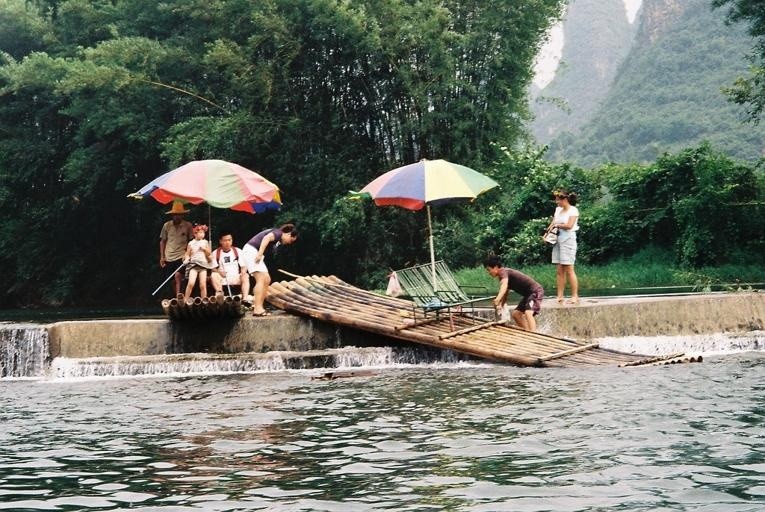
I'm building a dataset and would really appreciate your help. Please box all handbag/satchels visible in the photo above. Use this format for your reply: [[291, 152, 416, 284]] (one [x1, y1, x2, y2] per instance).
[[545, 226, 559, 245]]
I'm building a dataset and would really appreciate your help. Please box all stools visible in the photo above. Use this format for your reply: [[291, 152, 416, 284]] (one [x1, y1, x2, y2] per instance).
[[184, 277, 215, 298]]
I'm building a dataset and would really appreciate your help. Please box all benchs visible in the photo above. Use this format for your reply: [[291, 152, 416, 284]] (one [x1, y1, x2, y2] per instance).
[[394, 258, 502, 341]]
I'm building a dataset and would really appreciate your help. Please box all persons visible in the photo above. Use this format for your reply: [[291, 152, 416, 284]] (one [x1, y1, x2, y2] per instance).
[[482, 254, 545, 333], [209, 232, 252, 301], [543, 188, 581, 305], [184, 223, 211, 302], [159, 202, 194, 297], [241, 223, 300, 317]]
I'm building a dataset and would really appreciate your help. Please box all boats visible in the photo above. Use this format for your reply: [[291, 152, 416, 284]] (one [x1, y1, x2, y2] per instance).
[[157, 291, 263, 316], [262, 271, 704, 370]]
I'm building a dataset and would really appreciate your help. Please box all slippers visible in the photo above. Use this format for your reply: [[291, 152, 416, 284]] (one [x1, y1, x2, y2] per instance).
[[253, 310, 272, 317]]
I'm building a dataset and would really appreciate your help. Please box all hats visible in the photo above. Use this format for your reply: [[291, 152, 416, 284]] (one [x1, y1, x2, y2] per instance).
[[165, 202, 189, 214]]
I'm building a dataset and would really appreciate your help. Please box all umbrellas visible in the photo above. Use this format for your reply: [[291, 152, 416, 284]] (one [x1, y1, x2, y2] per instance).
[[127, 157, 283, 242], [345, 157, 501, 291]]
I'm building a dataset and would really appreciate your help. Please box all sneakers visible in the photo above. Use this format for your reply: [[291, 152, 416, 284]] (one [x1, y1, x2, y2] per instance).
[[557, 298, 579, 305]]
